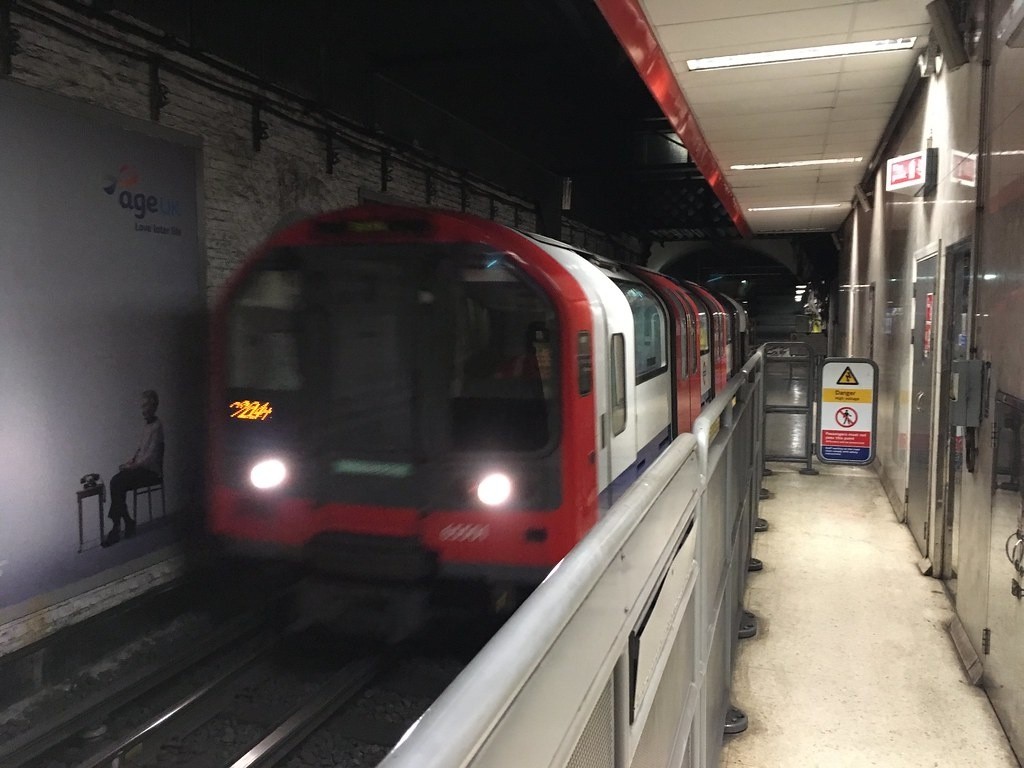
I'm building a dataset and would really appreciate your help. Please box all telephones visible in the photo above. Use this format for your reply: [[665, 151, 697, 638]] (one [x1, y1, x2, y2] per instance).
[[80, 473, 100, 489]]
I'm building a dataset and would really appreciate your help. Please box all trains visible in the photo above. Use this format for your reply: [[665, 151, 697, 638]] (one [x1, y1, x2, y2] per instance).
[[203, 199, 748, 626]]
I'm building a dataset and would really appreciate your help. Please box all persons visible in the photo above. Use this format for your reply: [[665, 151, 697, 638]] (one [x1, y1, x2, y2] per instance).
[[100, 389, 164, 548]]
